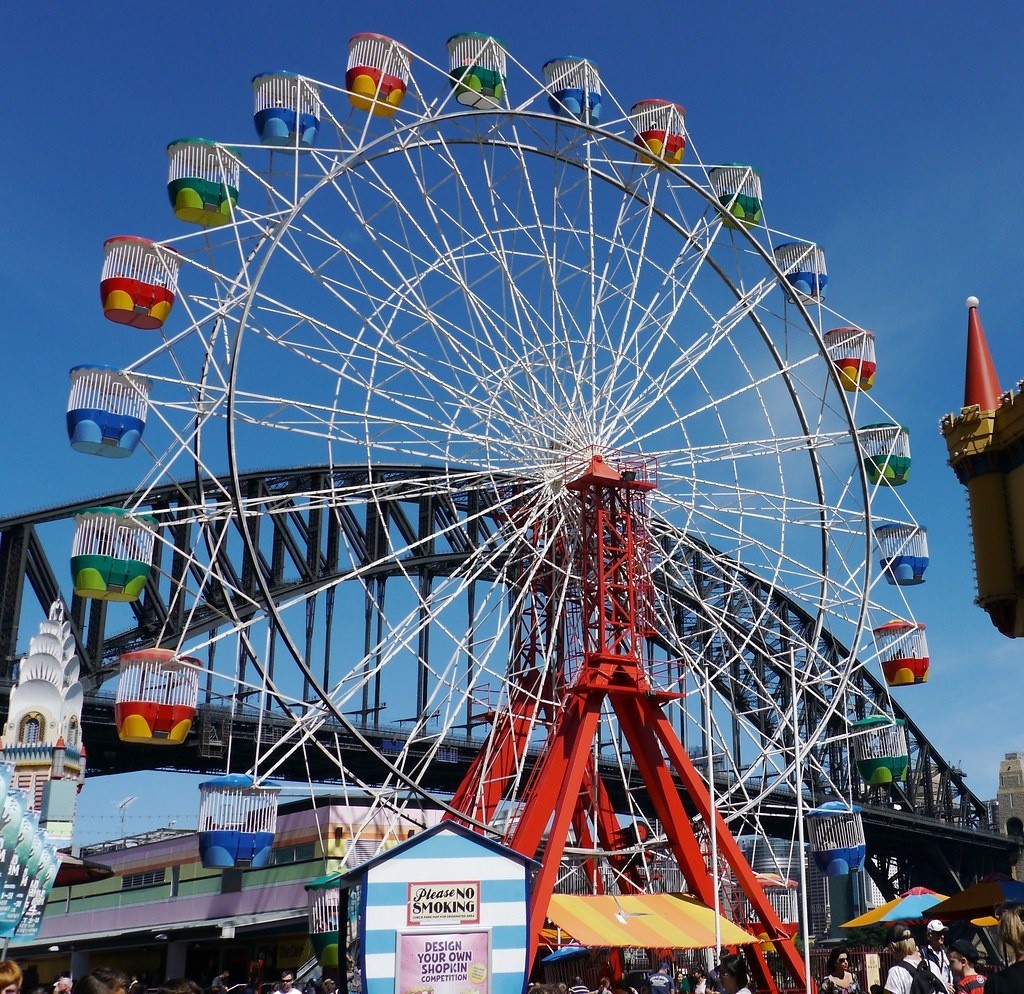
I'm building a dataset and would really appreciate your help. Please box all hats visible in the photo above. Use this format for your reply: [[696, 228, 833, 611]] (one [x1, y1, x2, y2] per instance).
[[659, 963, 668, 968], [950, 939, 979, 962], [883, 924, 913, 946], [927, 920, 948, 936]]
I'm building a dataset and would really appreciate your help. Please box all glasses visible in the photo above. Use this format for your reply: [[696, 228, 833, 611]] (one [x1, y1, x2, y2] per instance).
[[934, 931, 944, 936], [839, 958, 849, 963], [718, 971, 728, 978]]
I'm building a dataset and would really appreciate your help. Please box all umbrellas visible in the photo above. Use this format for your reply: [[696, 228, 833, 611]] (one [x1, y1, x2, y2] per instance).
[[838, 870, 1024, 968]]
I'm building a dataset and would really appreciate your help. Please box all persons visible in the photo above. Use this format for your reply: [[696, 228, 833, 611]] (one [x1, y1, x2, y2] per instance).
[[205, 817, 221, 830], [882, 918, 988, 994], [818, 945, 862, 994], [891, 647, 918, 660], [983, 906, 1024, 994], [0, 943, 363, 994], [529, 948, 756, 994]]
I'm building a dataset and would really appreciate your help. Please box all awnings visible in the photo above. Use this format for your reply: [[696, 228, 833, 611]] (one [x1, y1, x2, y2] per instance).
[[538, 889, 790, 962], [755, 873, 798, 891]]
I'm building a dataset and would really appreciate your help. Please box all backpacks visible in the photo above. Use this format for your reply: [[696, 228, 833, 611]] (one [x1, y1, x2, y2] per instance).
[[897, 956, 948, 994]]
